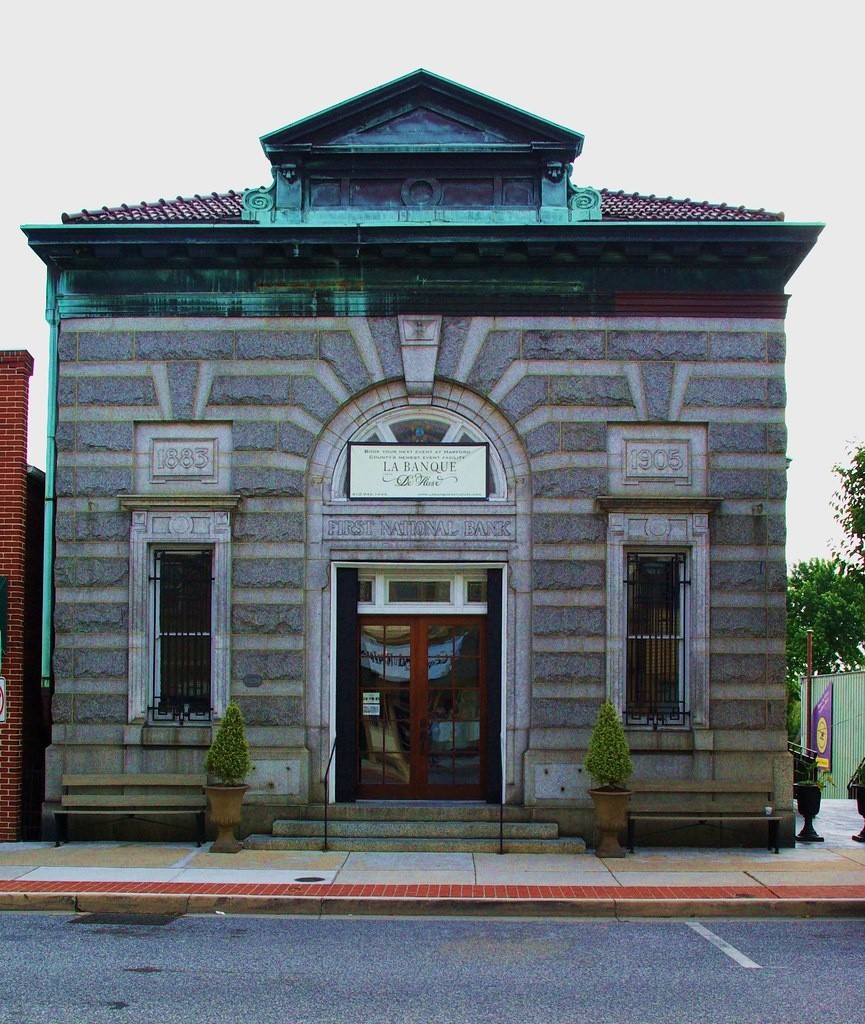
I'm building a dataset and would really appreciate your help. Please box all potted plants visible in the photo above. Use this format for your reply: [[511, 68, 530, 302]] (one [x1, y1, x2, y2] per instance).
[[852, 766, 865, 842], [202, 699, 250, 854], [584, 694, 634, 858], [792, 762, 836, 841]]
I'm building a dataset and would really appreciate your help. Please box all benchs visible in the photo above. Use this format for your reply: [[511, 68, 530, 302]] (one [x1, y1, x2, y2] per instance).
[[626, 781, 784, 854], [52, 773, 207, 847]]
